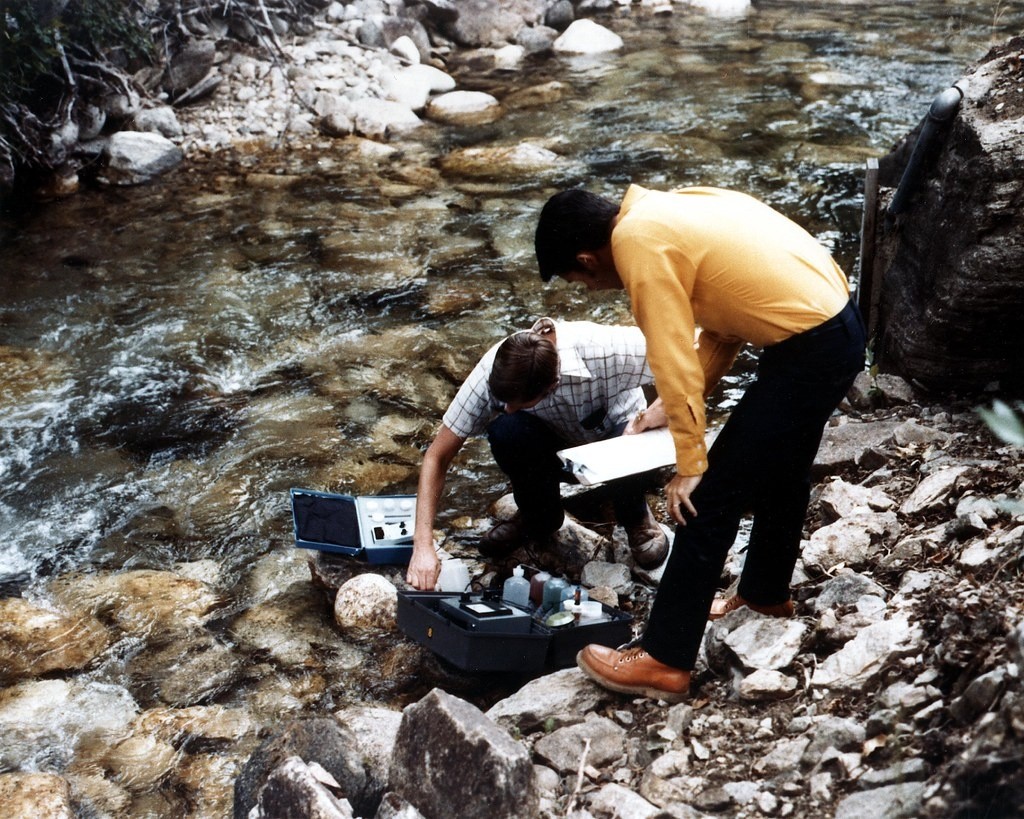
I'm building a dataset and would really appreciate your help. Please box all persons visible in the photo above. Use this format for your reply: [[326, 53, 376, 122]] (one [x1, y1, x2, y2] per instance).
[[531, 181, 865, 696], [407, 315, 670, 595]]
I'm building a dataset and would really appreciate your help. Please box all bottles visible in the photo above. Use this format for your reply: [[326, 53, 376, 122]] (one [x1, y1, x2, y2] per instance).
[[503, 568, 529, 605], [531, 568, 551, 603], [543, 573, 570, 612], [560, 577, 588, 613]]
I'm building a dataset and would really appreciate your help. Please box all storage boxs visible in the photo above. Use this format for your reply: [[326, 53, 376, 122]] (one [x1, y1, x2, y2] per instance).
[[398, 562, 635, 688], [290, 487, 416, 564]]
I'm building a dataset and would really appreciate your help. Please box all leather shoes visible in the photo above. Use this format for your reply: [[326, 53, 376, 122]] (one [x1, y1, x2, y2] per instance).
[[710, 595, 794, 621], [575, 643, 692, 705]]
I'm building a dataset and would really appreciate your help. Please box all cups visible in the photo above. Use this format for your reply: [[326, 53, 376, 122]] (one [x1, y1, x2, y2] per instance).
[[564, 600, 601, 617]]
